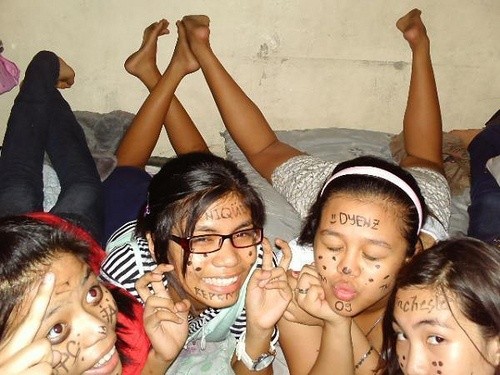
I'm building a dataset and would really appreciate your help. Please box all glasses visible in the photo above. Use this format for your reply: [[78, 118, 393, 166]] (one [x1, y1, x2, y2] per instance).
[[168, 223, 263, 254]]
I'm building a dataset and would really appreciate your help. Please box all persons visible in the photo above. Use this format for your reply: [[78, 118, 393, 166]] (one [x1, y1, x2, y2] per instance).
[[0, 49, 152, 375], [381, 99, 500, 375], [95, 19, 293, 375], [181, 7, 453, 375]]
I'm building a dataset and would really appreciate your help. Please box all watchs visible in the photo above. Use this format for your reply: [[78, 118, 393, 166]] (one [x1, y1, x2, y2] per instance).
[[235, 330, 278, 371]]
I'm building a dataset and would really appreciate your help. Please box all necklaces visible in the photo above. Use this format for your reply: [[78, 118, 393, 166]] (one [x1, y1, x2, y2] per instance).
[[364, 309, 385, 337]]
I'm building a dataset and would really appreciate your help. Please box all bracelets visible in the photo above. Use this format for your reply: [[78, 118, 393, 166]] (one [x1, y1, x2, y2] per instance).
[[353, 346, 374, 372]]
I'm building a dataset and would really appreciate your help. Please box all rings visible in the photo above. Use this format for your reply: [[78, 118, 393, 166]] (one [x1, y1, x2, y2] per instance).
[[297, 288, 309, 294]]
